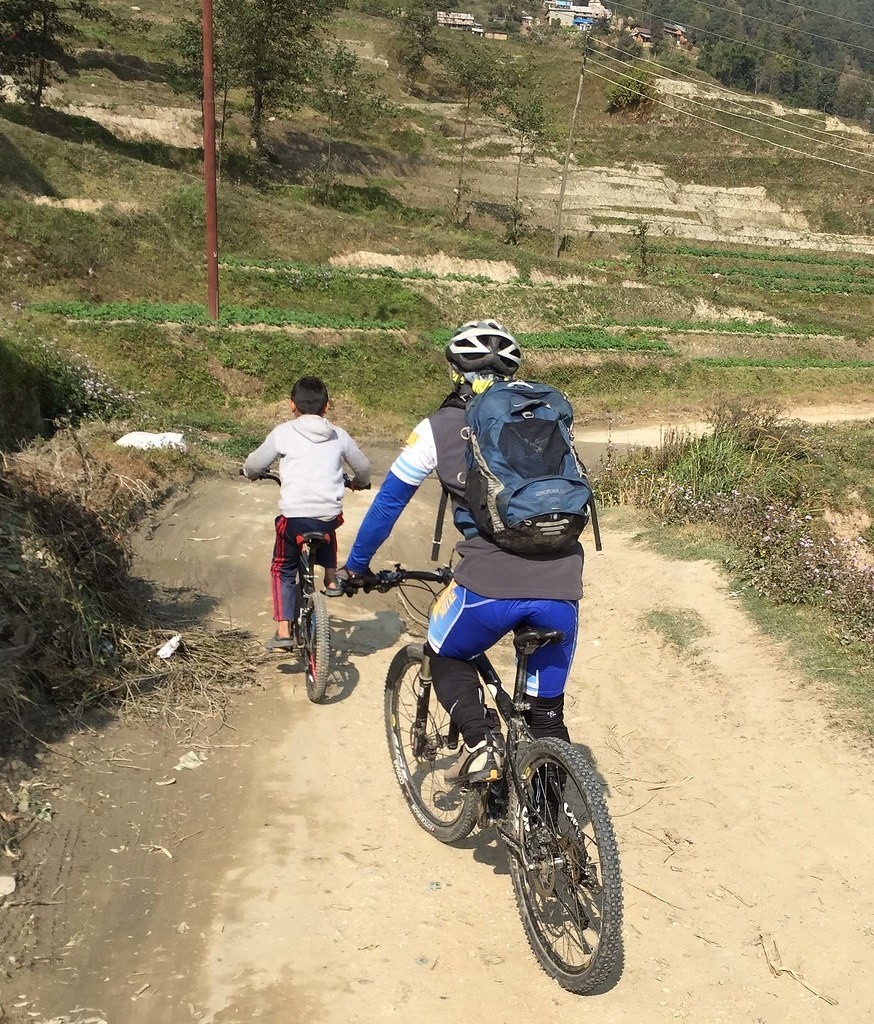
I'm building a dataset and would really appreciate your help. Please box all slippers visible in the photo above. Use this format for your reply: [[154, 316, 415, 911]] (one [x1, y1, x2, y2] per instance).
[[272, 630, 295, 647], [324, 576, 344, 597]]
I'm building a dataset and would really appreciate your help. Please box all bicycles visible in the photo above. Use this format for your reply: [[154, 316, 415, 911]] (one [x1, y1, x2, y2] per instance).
[[239, 469, 371, 705], [323, 570, 625, 997]]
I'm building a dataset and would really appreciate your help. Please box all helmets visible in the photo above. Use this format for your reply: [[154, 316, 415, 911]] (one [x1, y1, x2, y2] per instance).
[[445, 318, 522, 375]]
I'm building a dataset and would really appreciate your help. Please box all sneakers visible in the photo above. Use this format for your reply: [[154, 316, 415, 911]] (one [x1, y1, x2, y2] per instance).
[[444, 730, 503, 787]]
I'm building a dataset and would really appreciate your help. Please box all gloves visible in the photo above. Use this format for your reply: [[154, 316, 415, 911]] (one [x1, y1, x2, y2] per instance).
[[336, 565, 376, 599]]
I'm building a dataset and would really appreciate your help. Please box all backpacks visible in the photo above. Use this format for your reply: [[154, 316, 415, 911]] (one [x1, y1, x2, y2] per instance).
[[436, 379, 593, 558]]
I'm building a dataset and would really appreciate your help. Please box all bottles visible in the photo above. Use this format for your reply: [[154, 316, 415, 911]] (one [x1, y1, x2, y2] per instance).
[[486, 708, 506, 770]]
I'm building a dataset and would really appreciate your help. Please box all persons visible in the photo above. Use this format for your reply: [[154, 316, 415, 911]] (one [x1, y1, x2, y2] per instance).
[[242, 377, 370, 648], [334, 320, 584, 859]]
